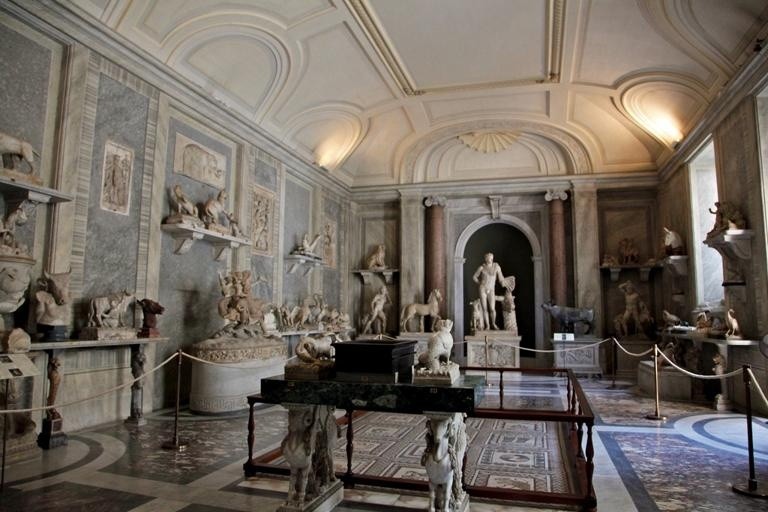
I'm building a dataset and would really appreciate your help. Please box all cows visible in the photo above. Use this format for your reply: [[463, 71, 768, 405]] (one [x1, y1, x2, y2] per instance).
[[541, 298, 597, 335], [86, 286, 135, 329], [35, 264, 73, 327]]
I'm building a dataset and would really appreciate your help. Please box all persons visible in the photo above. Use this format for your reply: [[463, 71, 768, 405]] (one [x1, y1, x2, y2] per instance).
[[203, 191, 232, 224], [619, 280, 642, 337], [362, 286, 392, 335], [473, 253, 505, 330], [709, 202, 722, 230], [175, 185, 200, 218]]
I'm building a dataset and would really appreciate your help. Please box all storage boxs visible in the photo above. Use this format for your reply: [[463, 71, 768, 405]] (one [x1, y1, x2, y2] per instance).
[[329, 337, 418, 374]]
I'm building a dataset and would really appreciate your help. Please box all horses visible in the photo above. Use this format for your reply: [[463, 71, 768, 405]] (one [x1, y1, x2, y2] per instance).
[[400, 287, 443, 332]]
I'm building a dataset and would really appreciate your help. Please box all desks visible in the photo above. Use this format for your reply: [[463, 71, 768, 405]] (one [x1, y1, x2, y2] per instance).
[[241, 372, 486, 496], [23, 332, 170, 450]]
